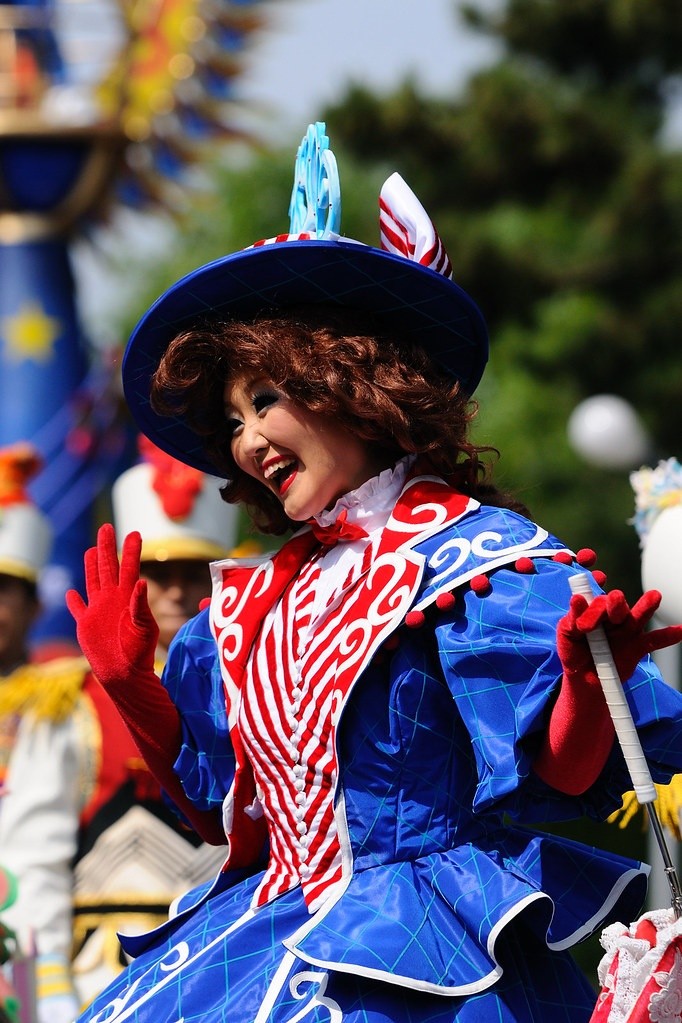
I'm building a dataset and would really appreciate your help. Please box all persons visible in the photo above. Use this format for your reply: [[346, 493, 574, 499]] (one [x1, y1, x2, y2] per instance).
[[65, 312, 681, 1023], [0, 462, 242, 1023]]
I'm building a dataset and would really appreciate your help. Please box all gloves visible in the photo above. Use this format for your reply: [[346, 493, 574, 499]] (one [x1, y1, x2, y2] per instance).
[[66, 524, 184, 754], [527, 590, 681, 796]]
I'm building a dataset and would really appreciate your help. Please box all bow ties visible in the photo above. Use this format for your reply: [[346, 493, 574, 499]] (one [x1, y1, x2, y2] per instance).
[[305, 508, 369, 545]]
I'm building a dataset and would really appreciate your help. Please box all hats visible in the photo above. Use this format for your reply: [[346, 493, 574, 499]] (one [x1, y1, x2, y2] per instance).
[[0, 440, 57, 581], [112, 461, 238, 559], [121, 122, 490, 481]]
